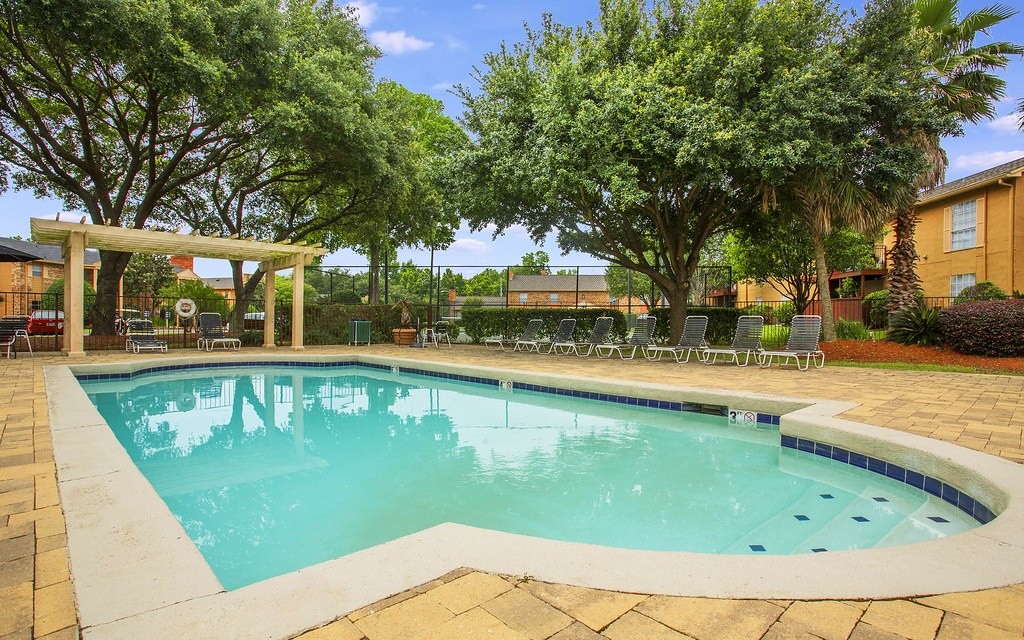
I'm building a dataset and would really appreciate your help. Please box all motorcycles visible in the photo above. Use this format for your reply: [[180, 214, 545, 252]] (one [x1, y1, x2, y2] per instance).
[[114, 313, 131, 335]]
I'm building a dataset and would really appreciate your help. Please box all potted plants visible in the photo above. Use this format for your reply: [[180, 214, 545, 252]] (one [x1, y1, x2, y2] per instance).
[[389, 299, 417, 344], [835, 278, 861, 298]]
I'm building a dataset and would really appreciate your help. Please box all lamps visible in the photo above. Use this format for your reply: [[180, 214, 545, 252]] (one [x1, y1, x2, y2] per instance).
[[582, 294, 585, 299]]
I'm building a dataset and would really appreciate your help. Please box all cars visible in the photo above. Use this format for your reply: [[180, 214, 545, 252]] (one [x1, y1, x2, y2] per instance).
[[27, 310, 64, 337]]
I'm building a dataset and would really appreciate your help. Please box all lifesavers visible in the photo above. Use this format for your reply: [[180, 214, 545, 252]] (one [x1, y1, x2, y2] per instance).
[[176, 299, 196, 317]]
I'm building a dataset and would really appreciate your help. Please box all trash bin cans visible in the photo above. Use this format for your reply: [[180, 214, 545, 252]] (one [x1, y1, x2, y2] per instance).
[[349, 319, 371, 347]]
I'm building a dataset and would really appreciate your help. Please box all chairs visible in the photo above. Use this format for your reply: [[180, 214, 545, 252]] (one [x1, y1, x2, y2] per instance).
[[0, 316, 34, 360], [758, 315, 825, 371], [126, 318, 169, 355], [486, 318, 543, 353], [648, 316, 710, 363], [554, 317, 614, 356], [513, 319, 576, 353], [595, 316, 658, 360], [197, 311, 242, 352], [702, 315, 766, 367], [422, 320, 452, 349]]
